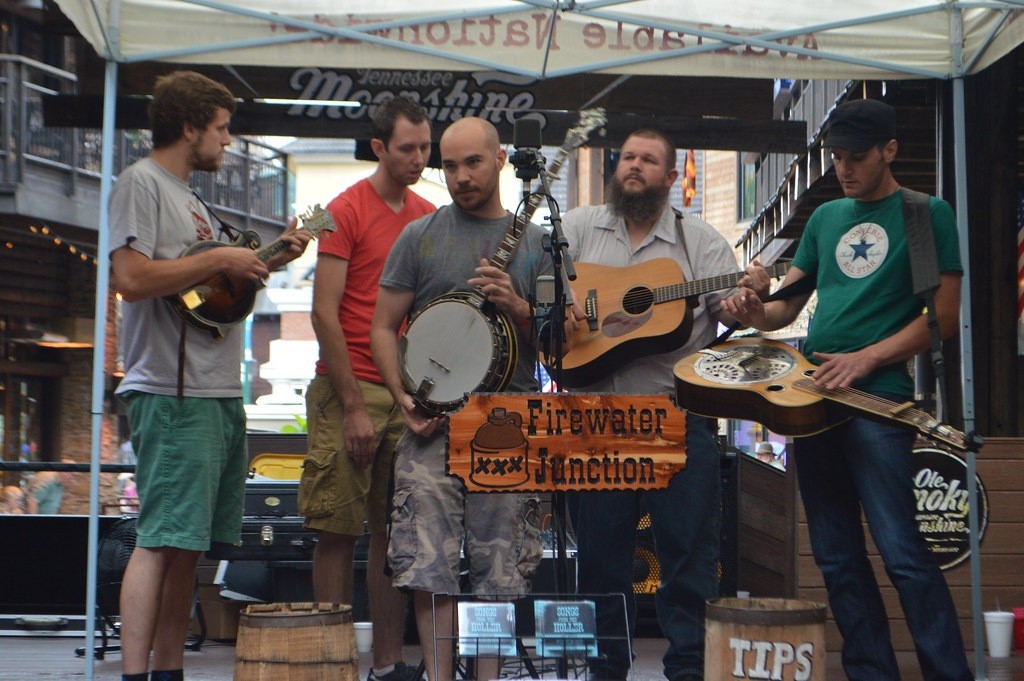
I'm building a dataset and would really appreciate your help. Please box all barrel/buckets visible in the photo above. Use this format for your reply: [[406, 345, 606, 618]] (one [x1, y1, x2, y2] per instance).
[[704, 598, 827, 681], [233, 602, 360, 681]]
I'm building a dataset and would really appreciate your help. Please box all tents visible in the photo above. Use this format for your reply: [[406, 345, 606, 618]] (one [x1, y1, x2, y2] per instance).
[[46, 0, 1023, 681]]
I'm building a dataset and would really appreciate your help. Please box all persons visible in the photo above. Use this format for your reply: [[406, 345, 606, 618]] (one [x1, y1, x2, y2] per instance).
[[109, 73, 313, 681], [551, 127, 770, 681], [367, 116, 574, 681], [719, 98, 975, 681], [0, 459, 139, 515], [297, 95, 439, 680]]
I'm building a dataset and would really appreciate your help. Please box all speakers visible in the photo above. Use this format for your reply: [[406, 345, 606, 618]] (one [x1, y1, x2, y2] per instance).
[[632, 451, 738, 637]]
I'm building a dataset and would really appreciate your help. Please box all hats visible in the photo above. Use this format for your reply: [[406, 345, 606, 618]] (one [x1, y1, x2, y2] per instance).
[[818, 99, 899, 153]]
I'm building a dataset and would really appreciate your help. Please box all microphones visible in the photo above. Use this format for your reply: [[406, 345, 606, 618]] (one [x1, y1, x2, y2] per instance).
[[514, 118, 542, 203]]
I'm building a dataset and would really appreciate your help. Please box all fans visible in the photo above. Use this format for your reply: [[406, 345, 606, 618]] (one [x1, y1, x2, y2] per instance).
[[76, 517, 207, 660]]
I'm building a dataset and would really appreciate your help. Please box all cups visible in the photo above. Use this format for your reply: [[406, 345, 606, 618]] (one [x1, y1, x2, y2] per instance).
[[1013, 609, 1024, 654], [984, 611, 1015, 658]]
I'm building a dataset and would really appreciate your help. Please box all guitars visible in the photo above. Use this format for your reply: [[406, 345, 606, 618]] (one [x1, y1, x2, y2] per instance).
[[671, 336, 984, 455], [539, 256, 791, 387], [168, 203, 339, 340], [398, 108, 608, 417]]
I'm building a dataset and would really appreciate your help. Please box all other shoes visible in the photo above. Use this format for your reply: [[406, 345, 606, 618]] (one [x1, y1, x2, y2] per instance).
[[367, 662, 426, 681]]
